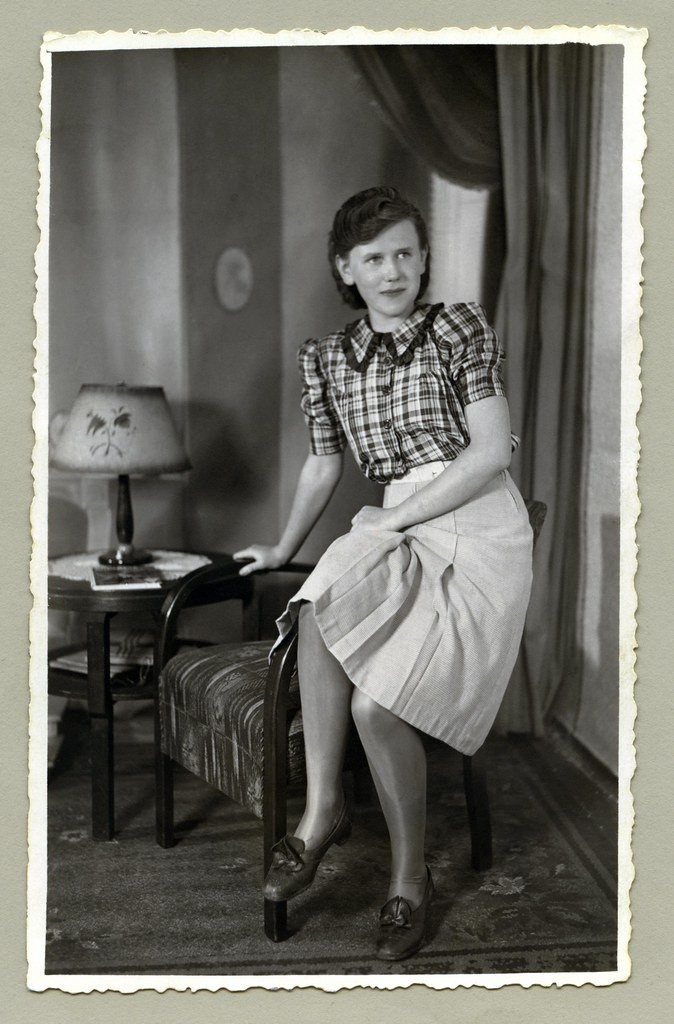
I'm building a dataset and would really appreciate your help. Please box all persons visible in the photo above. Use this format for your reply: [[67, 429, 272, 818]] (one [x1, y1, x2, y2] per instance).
[[233, 185, 534, 959]]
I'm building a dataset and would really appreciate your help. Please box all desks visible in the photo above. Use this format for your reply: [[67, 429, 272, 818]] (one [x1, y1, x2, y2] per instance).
[[48, 546, 265, 845]]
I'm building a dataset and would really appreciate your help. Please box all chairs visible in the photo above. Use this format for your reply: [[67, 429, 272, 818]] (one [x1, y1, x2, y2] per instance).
[[153, 498, 548, 945]]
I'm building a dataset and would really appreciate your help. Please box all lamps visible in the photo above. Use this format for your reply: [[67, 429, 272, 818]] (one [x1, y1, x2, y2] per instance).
[[51, 380, 194, 568]]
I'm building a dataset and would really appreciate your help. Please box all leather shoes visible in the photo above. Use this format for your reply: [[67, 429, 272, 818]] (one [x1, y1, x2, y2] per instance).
[[376, 864, 436, 961], [262, 796, 356, 903]]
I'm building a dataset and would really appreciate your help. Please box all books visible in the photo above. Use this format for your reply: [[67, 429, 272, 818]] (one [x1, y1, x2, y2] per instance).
[[88, 565, 162, 590], [49, 645, 154, 678]]
